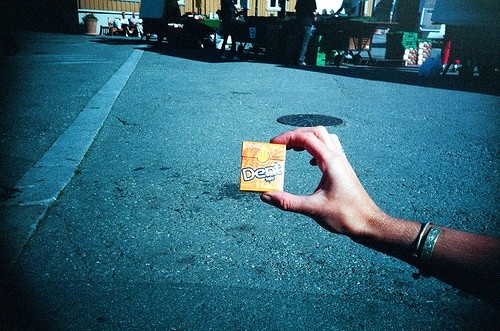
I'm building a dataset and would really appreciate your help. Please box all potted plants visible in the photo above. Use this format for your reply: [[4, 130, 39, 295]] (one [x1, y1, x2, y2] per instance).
[[82, 13, 98, 33]]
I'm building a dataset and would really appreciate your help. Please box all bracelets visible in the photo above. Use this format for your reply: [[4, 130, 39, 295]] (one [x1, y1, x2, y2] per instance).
[[419, 226, 442, 278], [412, 221, 433, 267]]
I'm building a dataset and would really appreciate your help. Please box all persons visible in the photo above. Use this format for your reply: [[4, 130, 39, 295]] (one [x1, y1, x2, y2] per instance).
[[294, 0, 317, 66], [259, 126, 500, 307], [217, 0, 241, 61], [121, 12, 133, 37], [129, 12, 144, 39]]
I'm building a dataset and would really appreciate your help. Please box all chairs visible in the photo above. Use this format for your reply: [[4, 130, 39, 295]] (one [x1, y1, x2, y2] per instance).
[[142, 18, 176, 52], [216, 10, 252, 53]]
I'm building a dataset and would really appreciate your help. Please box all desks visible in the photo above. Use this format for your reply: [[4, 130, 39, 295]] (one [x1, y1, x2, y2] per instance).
[[179, 17, 222, 50], [316, 14, 399, 66]]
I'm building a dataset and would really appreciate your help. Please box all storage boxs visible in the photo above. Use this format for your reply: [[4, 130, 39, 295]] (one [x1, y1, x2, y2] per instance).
[[239, 142, 288, 193], [386, 31, 420, 67], [417, 38, 432, 65]]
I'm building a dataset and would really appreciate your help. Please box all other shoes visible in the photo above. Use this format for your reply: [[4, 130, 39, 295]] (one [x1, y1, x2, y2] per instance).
[[440, 66, 445, 72], [234, 56, 240, 60], [451, 68, 457, 73], [221, 55, 228, 60], [296, 61, 310, 70]]
